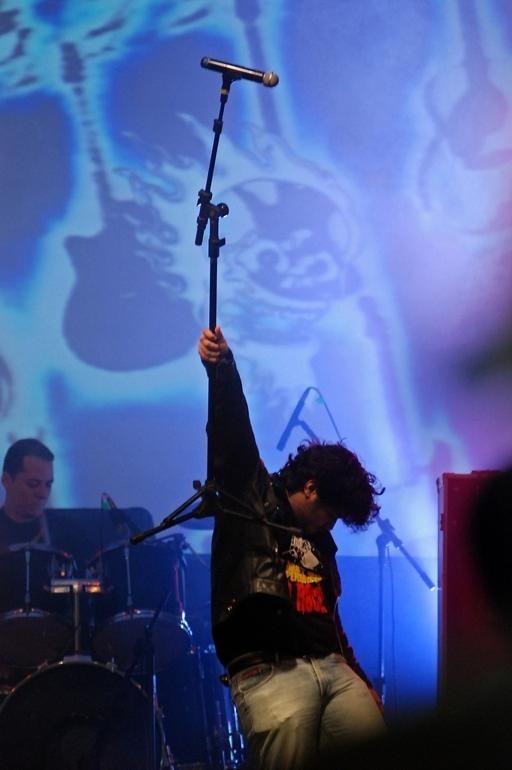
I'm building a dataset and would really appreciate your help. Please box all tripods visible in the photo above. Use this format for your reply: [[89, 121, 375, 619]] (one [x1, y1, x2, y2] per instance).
[[130, 78, 304, 545]]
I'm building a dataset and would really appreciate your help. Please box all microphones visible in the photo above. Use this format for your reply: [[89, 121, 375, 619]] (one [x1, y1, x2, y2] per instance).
[[277, 392, 308, 451], [200, 56, 279, 87], [106, 494, 128, 538]]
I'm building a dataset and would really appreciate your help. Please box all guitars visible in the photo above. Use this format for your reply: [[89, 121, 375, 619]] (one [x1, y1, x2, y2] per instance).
[[59, 40, 207, 371]]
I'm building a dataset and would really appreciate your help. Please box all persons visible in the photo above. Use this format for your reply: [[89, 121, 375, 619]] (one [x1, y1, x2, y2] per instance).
[[198, 325, 373, 770], [320, 468, 512, 770], [0, 439, 103, 613]]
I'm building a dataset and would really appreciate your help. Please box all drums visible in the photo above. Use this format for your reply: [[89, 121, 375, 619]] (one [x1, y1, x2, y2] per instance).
[[85, 538, 191, 674], [1, 655, 165, 769], [0, 542, 78, 668]]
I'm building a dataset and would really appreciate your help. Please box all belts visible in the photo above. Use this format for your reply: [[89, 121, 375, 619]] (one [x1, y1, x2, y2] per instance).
[[227, 652, 304, 678]]
